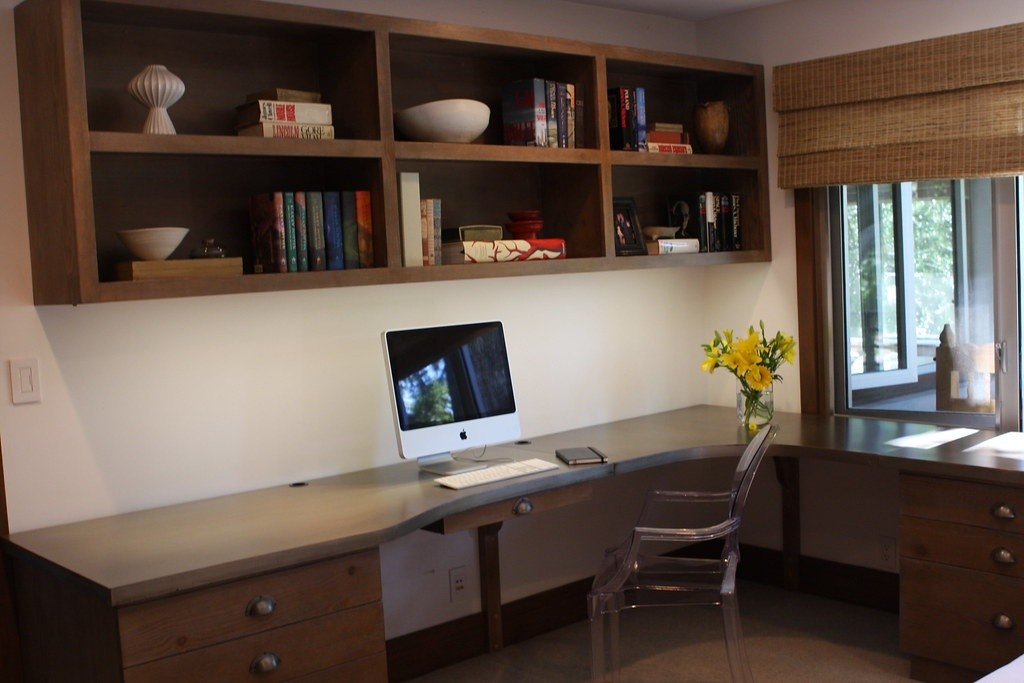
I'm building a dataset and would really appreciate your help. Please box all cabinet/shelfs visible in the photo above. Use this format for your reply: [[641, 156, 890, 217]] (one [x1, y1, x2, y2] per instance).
[[13, 0, 772, 305]]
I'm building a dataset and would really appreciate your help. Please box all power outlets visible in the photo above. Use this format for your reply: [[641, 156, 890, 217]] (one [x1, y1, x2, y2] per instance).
[[451, 566, 471, 604]]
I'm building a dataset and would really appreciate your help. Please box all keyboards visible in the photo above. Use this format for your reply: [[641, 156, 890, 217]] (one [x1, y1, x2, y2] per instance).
[[433, 458, 558, 491]]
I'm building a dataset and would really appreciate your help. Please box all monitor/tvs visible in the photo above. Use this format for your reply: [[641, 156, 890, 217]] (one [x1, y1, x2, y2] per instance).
[[381, 320, 522, 475]]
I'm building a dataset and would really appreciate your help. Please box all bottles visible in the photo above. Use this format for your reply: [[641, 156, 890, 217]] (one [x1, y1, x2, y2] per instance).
[[187, 238, 224, 258]]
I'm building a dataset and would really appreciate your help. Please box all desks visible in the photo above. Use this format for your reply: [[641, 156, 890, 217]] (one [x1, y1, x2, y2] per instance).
[[0, 405, 1024, 683]]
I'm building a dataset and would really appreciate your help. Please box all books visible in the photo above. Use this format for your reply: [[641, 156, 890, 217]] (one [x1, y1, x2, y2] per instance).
[[234, 99, 332, 128], [238, 122, 334, 140], [555, 446, 608, 465], [501, 78, 588, 147], [248, 184, 374, 273], [246, 88, 321, 104], [607, 87, 695, 153], [400, 172, 567, 267], [646, 191, 739, 256]]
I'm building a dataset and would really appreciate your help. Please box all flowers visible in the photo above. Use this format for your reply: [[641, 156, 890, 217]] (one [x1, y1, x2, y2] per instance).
[[701, 319, 797, 430]]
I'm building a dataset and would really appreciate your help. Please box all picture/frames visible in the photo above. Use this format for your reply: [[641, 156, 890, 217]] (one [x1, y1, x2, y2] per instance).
[[612, 196, 648, 256]]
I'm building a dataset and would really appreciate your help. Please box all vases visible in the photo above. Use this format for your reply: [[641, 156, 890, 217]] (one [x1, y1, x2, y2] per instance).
[[736, 378, 775, 424]]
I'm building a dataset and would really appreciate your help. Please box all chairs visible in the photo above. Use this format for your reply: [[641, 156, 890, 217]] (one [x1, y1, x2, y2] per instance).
[[588, 423, 779, 683]]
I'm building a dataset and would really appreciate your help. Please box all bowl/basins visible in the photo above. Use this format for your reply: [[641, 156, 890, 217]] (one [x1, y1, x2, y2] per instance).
[[119, 225, 190, 262], [396, 99, 492, 143]]
